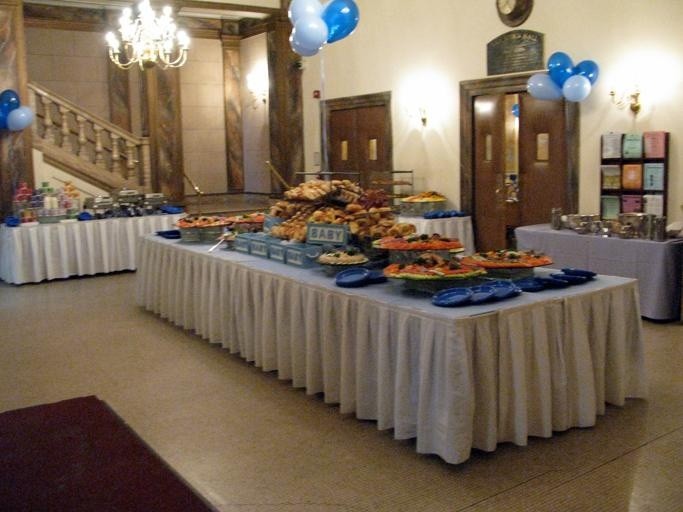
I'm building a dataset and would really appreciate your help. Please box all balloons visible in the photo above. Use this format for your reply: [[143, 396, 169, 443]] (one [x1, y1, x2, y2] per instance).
[[286, 1, 360, 57], [525, 73, 561, 100], [573, 60, 599, 87], [547, 51, 573, 87], [562, 74, 592, 102], [511, 104, 519, 117], [0, 90, 34, 132]]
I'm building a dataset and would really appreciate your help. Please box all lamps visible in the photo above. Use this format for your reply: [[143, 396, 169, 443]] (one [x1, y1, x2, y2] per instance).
[[606, 73, 640, 113], [102, 0, 193, 72]]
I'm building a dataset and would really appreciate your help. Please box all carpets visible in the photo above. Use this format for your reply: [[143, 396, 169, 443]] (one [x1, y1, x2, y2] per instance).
[[0, 394, 220, 512]]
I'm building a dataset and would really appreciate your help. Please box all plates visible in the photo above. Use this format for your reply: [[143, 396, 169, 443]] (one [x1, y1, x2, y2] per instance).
[[421, 208, 466, 219], [400, 197, 447, 204], [152, 215, 265, 239], [75, 203, 184, 221], [317, 233, 600, 309]]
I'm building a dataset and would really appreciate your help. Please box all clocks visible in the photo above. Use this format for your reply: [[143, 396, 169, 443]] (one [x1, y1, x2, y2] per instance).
[[496, 0, 533, 27]]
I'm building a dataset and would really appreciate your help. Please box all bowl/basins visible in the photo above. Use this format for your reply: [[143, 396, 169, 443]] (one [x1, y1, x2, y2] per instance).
[[34, 214, 66, 224], [548, 206, 681, 242], [4, 217, 19, 226]]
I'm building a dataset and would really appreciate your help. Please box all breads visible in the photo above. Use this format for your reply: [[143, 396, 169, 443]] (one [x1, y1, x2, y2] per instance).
[[269, 178, 417, 242]]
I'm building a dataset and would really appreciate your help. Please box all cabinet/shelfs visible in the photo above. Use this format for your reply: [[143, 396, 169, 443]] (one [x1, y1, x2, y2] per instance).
[[367, 169, 414, 209]]
